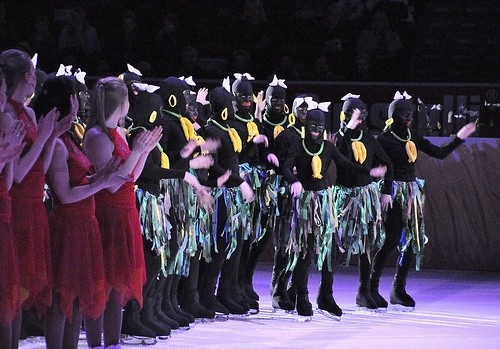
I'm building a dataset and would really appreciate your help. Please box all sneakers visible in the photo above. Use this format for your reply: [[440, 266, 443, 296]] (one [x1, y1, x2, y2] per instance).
[[118, 287, 261, 349], [272, 294, 295, 315], [295, 299, 313, 322], [354, 293, 377, 315], [316, 295, 343, 320], [389, 291, 415, 313], [370, 291, 389, 312]]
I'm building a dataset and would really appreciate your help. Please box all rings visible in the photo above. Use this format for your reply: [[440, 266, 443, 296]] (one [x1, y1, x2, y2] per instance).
[[15, 134, 19, 137]]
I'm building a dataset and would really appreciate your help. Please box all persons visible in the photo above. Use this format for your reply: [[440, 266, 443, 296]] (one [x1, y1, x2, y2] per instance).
[[34, 75, 134, 349], [82, 78, 163, 348], [372, 99, 477, 308], [31, 0, 416, 102], [0, 68, 26, 349], [121, 77, 394, 338], [0, 48, 76, 349]]
